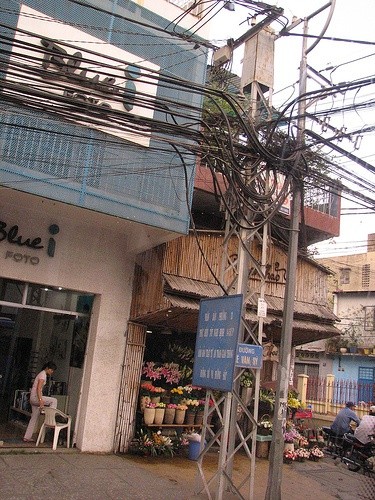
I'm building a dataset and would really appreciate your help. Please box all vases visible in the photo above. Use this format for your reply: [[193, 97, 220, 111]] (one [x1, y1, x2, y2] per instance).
[[284, 443, 294, 454], [164, 409, 175, 425], [154, 409, 165, 425], [144, 408, 155, 424], [260, 425, 270, 436], [195, 411, 204, 425], [175, 410, 185, 425], [184, 411, 196, 425], [242, 387, 253, 406]]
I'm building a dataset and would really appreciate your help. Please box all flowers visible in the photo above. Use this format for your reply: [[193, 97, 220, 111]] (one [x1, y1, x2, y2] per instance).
[[128, 343, 324, 464]]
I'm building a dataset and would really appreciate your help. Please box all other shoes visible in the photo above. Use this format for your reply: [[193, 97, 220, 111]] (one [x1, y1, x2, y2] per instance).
[[24, 438, 35, 442]]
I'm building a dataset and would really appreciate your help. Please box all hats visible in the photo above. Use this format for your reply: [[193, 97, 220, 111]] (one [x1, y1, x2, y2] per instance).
[[369, 405, 375, 413], [346, 401, 354, 407]]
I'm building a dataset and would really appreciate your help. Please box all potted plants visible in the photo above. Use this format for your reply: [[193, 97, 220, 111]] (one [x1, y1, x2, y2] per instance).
[[326, 337, 349, 354]]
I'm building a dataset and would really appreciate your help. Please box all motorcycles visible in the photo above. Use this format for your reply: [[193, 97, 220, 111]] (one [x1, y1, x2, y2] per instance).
[[320, 425, 375, 472]]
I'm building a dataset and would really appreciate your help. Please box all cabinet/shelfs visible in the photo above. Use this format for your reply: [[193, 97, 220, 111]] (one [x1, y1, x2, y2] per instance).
[[9, 393, 69, 435]]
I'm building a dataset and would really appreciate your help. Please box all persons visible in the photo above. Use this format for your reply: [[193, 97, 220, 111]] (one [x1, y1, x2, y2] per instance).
[[331, 401, 360, 436], [23, 361, 57, 442], [354, 406, 375, 444]]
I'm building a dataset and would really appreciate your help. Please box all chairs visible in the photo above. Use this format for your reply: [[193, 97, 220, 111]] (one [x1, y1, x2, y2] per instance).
[[36, 407, 71, 449]]
